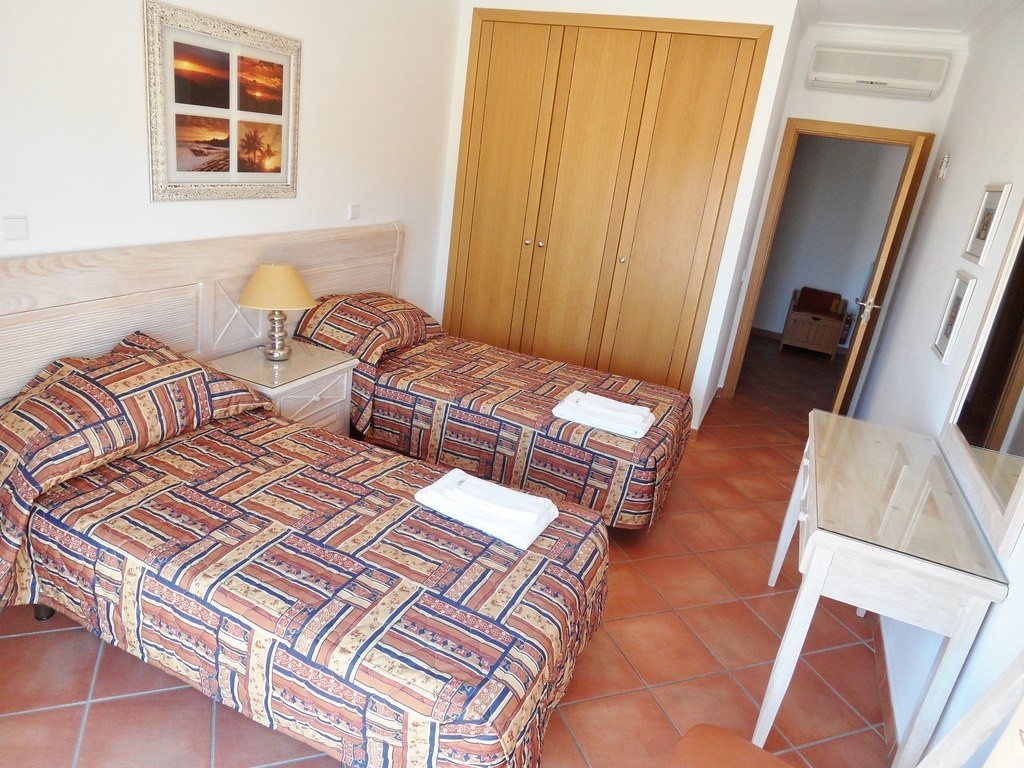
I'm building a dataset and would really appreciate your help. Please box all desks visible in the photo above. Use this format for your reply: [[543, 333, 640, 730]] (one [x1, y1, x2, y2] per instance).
[[752, 408, 1010, 768]]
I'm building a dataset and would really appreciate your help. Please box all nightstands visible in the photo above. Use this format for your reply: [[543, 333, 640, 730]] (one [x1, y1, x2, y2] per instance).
[[201, 337, 361, 438]]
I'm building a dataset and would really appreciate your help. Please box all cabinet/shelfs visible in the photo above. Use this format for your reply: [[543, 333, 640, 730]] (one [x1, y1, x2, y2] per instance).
[[442, 7, 774, 396], [779, 289, 849, 360]]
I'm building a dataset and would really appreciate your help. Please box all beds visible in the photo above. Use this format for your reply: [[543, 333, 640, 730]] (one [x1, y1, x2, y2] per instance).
[[294, 291, 694, 534], [0, 330, 611, 768]]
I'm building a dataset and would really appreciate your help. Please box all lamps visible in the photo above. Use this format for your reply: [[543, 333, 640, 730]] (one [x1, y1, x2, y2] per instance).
[[237, 262, 317, 361]]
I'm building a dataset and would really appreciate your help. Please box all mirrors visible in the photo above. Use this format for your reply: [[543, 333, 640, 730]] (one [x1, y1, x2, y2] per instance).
[[938, 200, 1024, 559]]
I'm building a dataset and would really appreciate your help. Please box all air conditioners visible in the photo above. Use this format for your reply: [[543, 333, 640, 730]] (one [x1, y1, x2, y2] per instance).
[[804, 42, 950, 102]]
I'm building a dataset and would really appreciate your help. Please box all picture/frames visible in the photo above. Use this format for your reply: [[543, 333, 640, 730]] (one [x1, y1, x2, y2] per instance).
[[144, 0, 302, 203], [961, 182, 1013, 270], [930, 269, 978, 366]]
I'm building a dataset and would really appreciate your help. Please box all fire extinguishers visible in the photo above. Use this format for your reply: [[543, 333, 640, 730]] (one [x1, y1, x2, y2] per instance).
[[839, 313, 855, 345]]
